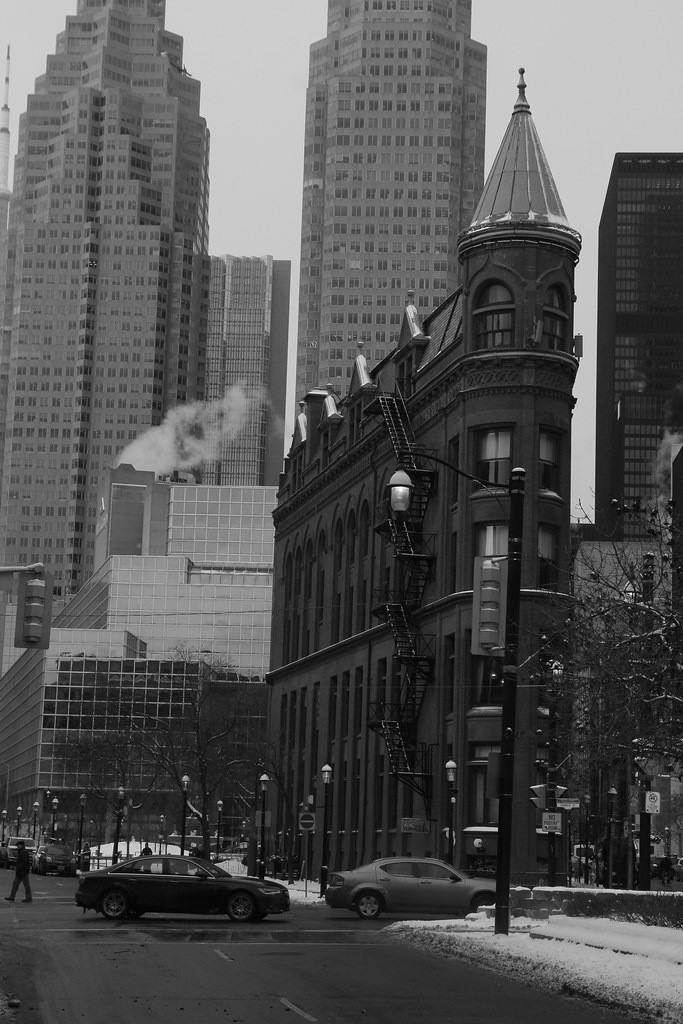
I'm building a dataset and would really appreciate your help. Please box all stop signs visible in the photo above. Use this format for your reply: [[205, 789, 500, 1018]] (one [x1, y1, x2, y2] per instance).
[[297, 813, 315, 831]]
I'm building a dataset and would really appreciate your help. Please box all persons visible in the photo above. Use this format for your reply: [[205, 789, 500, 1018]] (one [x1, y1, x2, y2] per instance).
[[84, 843, 90, 853], [4, 840, 33, 902], [661, 856, 674, 884], [73, 838, 80, 852], [140, 843, 152, 870]]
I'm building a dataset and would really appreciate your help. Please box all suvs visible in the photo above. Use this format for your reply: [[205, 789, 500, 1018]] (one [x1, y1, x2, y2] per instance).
[[0, 836, 38, 870]]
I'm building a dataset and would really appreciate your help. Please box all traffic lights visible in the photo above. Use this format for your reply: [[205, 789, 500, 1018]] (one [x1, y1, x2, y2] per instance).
[[528, 783, 547, 810], [556, 783, 567, 799]]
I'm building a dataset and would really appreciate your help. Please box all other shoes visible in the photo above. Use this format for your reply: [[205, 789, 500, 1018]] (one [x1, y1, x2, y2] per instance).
[[4, 897, 15, 901], [23, 899, 33, 903]]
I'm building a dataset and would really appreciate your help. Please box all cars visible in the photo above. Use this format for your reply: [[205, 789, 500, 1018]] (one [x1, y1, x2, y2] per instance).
[[32, 846, 47, 874], [326, 858, 495, 921], [74, 854, 291, 921], [38, 845, 77, 877]]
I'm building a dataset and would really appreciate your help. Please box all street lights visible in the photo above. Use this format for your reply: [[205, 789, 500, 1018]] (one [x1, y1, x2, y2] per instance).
[[64, 814, 68, 841], [258, 770, 270, 881], [76, 792, 87, 865], [179, 772, 190, 856], [320, 762, 333, 899], [159, 813, 164, 856], [241, 820, 247, 843], [606, 784, 618, 889], [1, 809, 7, 846], [50, 795, 60, 839], [445, 759, 457, 870], [90, 819, 93, 847], [114, 786, 125, 865], [582, 790, 593, 886], [665, 824, 670, 880], [16, 806, 23, 837], [32, 801, 40, 840], [388, 449, 525, 939], [216, 799, 223, 861]]
